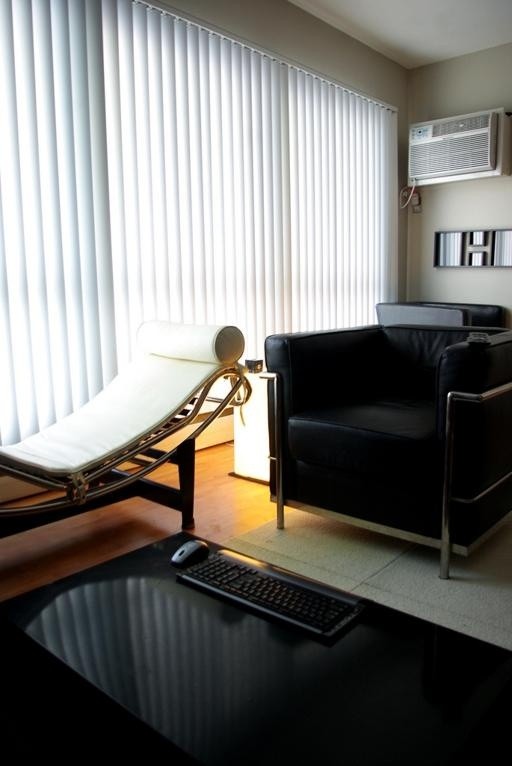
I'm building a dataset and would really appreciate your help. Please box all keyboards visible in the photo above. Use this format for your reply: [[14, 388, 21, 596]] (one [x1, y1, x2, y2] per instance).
[[176, 549, 369, 645]]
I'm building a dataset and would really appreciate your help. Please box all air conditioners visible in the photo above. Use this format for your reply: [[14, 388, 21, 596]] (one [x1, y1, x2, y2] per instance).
[[407, 106, 512, 187]]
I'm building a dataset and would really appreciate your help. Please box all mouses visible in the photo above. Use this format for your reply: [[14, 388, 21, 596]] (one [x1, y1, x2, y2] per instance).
[[170, 536, 209, 566]]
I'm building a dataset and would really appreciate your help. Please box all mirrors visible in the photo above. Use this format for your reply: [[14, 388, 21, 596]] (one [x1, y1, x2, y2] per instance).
[[433, 231, 512, 267]]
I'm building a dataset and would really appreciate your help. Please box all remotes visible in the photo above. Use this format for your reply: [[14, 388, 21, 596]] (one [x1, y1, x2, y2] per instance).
[[467, 332, 491, 347]]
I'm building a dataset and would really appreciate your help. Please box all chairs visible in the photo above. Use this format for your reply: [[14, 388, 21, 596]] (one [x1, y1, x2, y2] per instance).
[[376, 301, 505, 327], [264, 324, 511, 579], [0, 321, 251, 540]]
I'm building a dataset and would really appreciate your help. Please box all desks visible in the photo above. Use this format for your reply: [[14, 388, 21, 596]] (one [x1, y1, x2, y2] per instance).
[[232, 366, 274, 487], [1, 531, 511, 766]]
